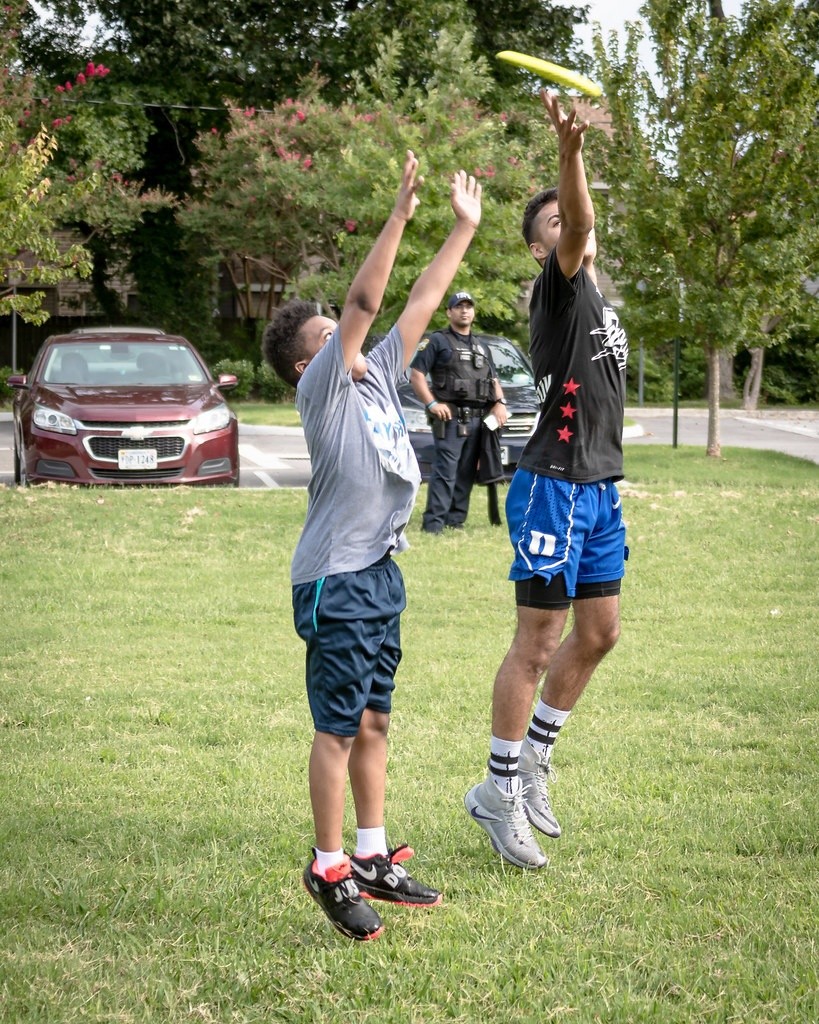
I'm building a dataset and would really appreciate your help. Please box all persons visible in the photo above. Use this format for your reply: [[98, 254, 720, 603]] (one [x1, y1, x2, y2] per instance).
[[462, 86, 634, 873], [408, 292, 509, 536], [257, 148, 484, 945]]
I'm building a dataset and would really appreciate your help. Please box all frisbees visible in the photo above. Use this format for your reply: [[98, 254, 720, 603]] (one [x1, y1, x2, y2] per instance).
[[493, 50, 601, 98]]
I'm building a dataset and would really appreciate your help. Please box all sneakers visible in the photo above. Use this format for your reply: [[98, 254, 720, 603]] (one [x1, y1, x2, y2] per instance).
[[517, 743, 565, 840], [300, 849, 385, 942], [347, 845, 446, 910], [463, 775, 551, 870]]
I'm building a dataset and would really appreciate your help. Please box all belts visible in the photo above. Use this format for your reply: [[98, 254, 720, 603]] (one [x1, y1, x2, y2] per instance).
[[443, 406, 490, 419]]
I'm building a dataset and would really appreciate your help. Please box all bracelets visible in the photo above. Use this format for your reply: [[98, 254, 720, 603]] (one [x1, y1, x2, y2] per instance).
[[427, 399, 439, 410]]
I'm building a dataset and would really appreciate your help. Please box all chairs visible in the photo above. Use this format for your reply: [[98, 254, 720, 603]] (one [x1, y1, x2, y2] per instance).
[[57, 353, 92, 387], [137, 352, 181, 385]]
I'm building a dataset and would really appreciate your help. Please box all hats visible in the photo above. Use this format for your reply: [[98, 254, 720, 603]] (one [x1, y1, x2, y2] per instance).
[[446, 293, 478, 308]]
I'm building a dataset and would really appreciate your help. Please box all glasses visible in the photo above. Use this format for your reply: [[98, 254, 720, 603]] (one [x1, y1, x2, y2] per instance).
[[455, 304, 475, 311]]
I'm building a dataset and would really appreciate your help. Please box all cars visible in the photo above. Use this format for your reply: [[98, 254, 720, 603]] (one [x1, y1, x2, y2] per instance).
[[6, 333, 239, 488], [394, 333, 541, 482]]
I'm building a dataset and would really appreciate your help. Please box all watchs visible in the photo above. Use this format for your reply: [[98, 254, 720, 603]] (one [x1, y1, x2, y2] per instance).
[[493, 397, 508, 406]]
